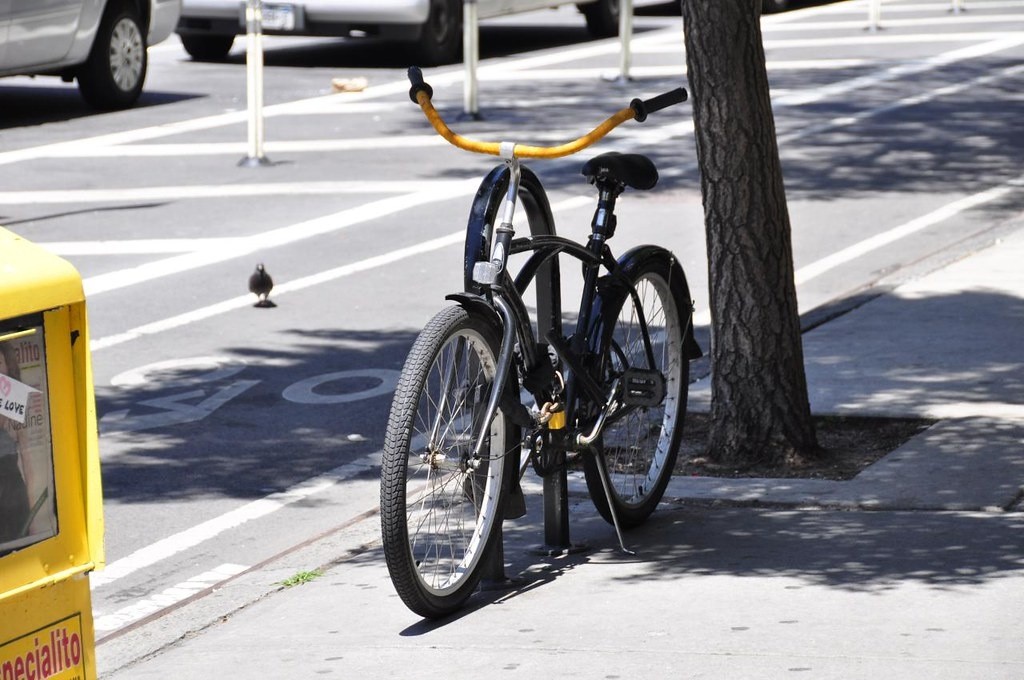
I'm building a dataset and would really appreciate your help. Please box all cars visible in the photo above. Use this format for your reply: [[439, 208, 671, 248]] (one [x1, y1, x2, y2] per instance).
[[0, 0, 182, 115], [174, 0, 620, 68]]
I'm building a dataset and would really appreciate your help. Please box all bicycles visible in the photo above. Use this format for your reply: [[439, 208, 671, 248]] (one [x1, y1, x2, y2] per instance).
[[379, 63, 705, 622]]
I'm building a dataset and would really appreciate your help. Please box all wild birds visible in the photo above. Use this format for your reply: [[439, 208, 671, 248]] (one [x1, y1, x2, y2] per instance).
[[248, 262, 273, 307]]
